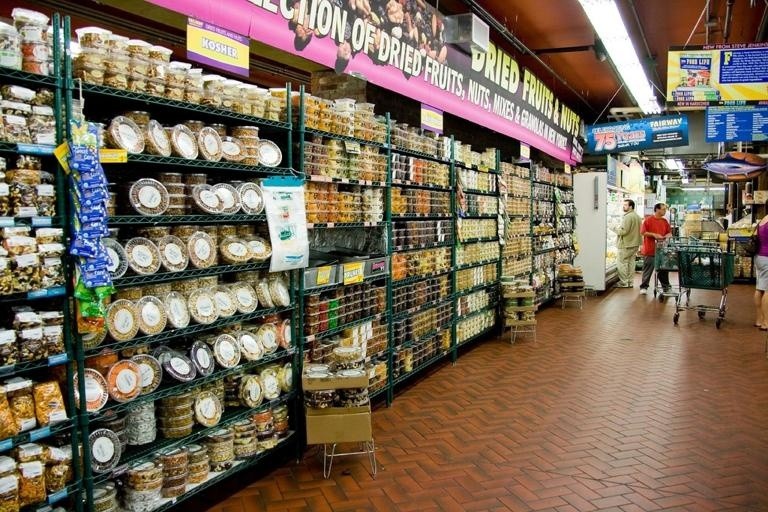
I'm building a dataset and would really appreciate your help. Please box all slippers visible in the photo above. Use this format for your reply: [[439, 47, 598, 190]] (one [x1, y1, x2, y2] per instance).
[[753, 322, 768, 331]]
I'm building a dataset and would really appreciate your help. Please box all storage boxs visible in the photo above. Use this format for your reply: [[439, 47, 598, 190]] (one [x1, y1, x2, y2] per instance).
[[562, 276, 585, 296], [503, 291, 538, 327], [302, 359, 371, 446]]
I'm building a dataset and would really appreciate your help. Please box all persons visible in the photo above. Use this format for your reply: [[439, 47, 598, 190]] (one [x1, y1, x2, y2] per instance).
[[610, 199, 642, 288], [753, 199, 768, 331], [639, 203, 672, 295]]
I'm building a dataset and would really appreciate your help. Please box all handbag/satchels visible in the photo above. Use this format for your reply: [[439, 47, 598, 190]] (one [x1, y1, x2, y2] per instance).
[[745, 235, 758, 256]]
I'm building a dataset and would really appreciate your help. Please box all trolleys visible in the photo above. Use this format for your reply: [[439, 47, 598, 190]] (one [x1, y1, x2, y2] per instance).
[[653, 236, 698, 307], [672, 245, 734, 330]]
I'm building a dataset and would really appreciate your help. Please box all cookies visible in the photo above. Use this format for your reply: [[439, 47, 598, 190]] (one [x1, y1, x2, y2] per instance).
[[71, 31, 282, 121]]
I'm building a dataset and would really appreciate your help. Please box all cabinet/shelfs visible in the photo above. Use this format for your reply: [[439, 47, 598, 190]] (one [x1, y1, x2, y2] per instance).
[[437, 129, 502, 359], [57, 12, 302, 512], [498, 152, 581, 335], [1, 11, 95, 511], [291, 81, 389, 429], [376, 112, 459, 400]]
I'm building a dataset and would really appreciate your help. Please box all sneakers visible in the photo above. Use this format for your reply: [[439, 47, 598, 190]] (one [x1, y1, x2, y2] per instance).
[[640, 288, 647, 295], [613, 282, 633, 288]]
